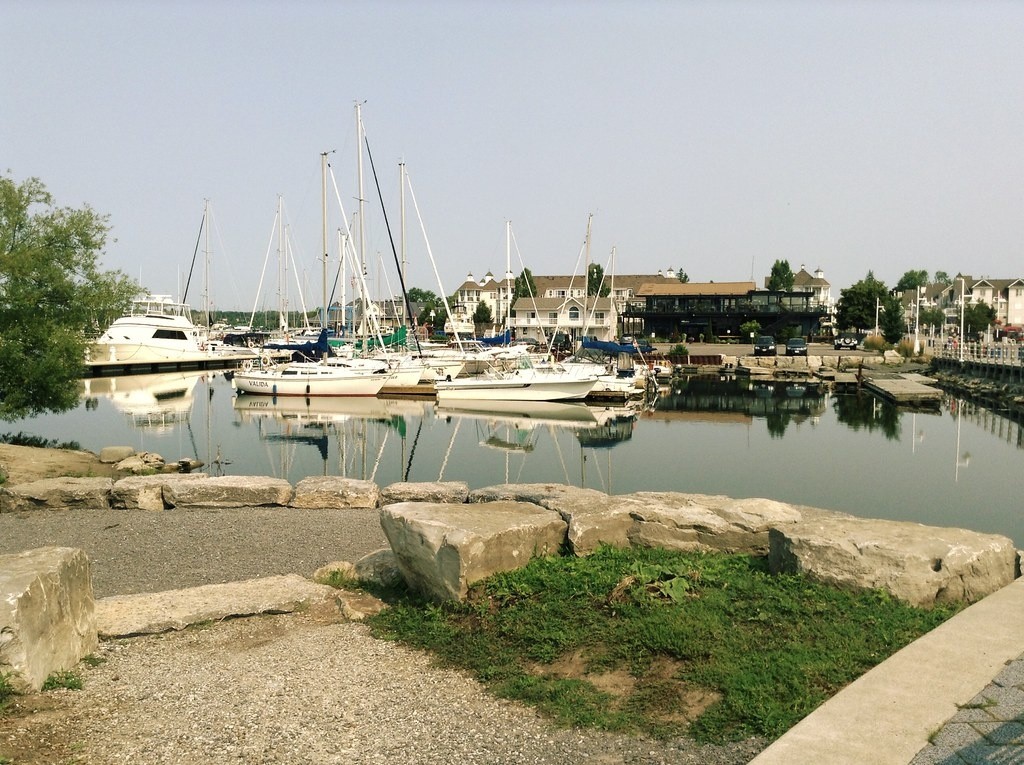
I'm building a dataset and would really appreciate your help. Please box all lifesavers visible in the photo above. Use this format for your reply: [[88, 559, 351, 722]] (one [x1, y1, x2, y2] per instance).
[[248, 342, 255, 348], [261, 356, 270, 367], [241, 361, 253, 372], [953, 340, 959, 349]]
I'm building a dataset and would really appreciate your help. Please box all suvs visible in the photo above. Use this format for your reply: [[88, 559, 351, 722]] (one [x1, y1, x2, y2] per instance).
[[754, 336, 778, 356], [834, 331, 858, 350]]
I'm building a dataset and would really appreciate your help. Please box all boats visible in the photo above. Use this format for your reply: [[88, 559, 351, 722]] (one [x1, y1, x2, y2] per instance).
[[82, 261, 264, 376], [71, 361, 243, 436]]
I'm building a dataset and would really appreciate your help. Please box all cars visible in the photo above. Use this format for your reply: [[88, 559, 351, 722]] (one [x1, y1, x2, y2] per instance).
[[786, 337, 809, 356]]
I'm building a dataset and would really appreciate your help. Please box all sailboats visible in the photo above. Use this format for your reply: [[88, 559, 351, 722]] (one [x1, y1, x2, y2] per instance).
[[578, 409, 639, 494], [232, 392, 438, 483], [435, 397, 613, 485], [177, 98, 675, 426]]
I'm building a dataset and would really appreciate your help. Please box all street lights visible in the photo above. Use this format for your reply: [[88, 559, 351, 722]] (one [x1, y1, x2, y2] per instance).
[[907, 299, 917, 317], [957, 278, 965, 363], [996, 290, 1006, 343], [955, 396, 972, 484], [875, 297, 886, 338], [911, 412, 924, 457], [913, 286, 932, 356]]
